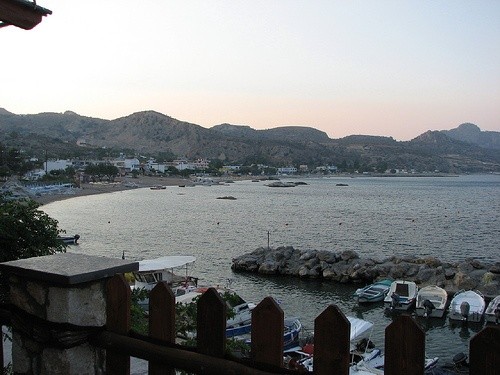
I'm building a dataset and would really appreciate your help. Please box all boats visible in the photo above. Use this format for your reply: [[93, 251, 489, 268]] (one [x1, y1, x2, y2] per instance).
[[415, 285, 448, 319], [48, 233, 80, 245], [150, 185, 166, 190], [382, 279, 418, 311], [448, 286, 500, 325], [354, 278, 394, 304], [130, 256, 439, 375]]
